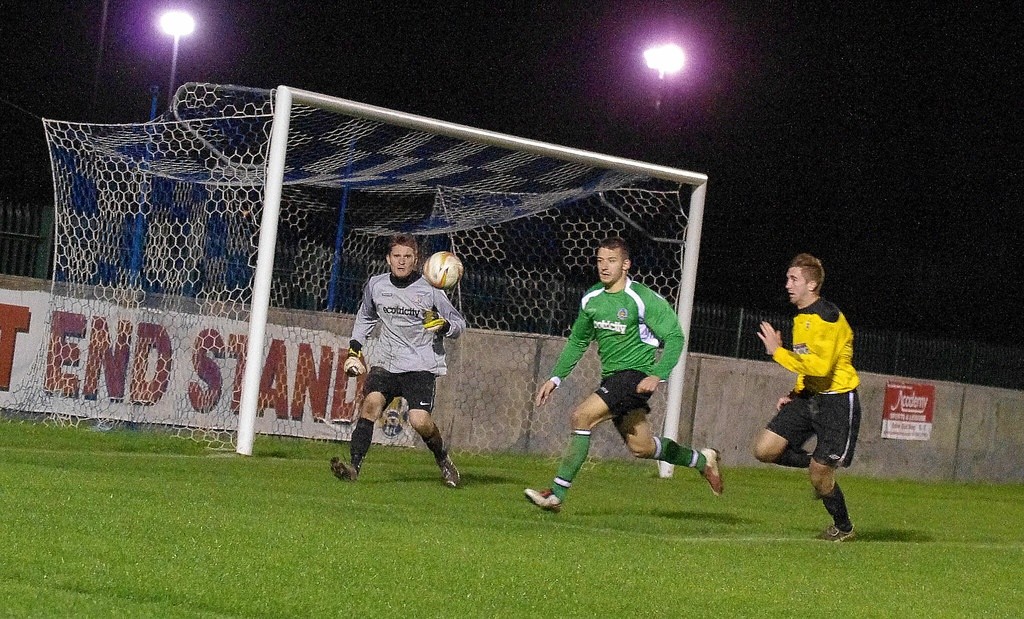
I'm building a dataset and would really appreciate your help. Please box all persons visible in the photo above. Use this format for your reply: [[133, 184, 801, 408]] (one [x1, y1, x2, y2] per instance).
[[753, 254, 862, 543], [523, 238, 722, 513], [330, 233, 467, 488]]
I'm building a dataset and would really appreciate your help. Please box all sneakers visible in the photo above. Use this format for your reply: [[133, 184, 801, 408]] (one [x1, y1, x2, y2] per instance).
[[436, 453, 460, 487], [814, 486, 823, 500], [701, 447, 724, 496], [524, 488, 561, 513], [331, 456, 357, 481], [819, 524, 856, 542]]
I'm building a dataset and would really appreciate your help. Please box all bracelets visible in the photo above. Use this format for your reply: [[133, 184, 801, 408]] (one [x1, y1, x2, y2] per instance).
[[549, 376, 561, 391]]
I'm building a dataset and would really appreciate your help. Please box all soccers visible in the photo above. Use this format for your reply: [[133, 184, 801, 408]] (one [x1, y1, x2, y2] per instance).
[[423, 250, 464, 290]]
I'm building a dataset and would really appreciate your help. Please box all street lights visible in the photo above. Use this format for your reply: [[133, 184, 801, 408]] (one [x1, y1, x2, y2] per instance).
[[158, 10, 196, 116], [643, 41, 684, 129]]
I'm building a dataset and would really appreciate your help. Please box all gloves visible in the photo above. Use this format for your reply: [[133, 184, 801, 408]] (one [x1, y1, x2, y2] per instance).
[[421, 312, 449, 333], [343, 348, 367, 377]]
